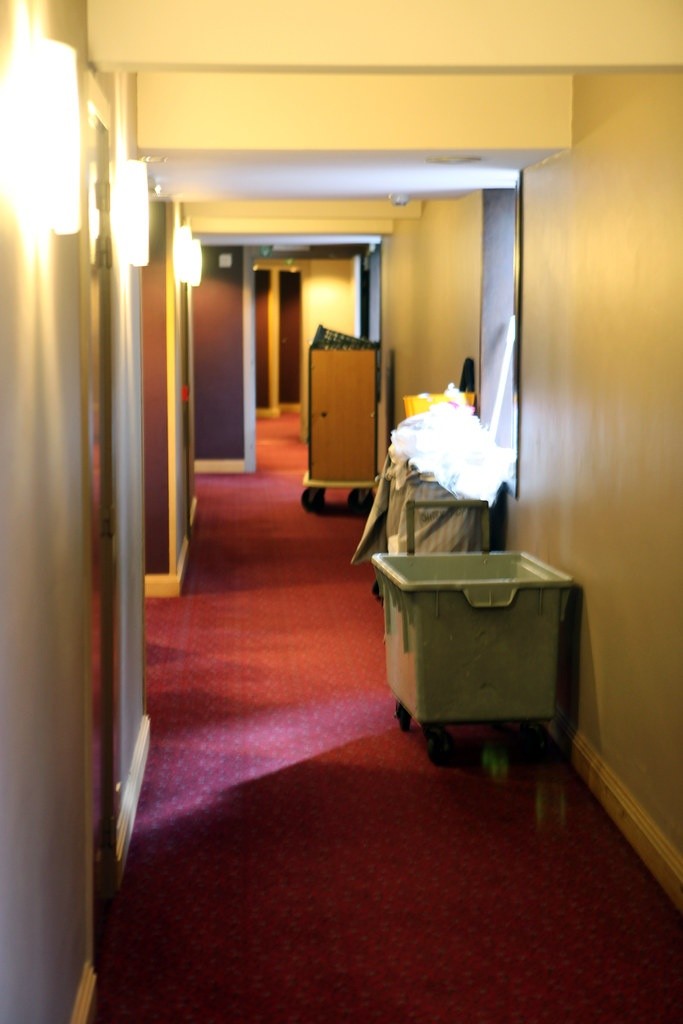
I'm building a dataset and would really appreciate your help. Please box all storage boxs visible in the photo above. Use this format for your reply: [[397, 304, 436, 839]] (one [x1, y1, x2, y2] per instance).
[[372, 550, 573, 766]]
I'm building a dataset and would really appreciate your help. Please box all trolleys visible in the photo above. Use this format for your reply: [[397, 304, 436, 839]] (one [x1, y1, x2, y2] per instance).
[[303, 340, 379, 511]]
